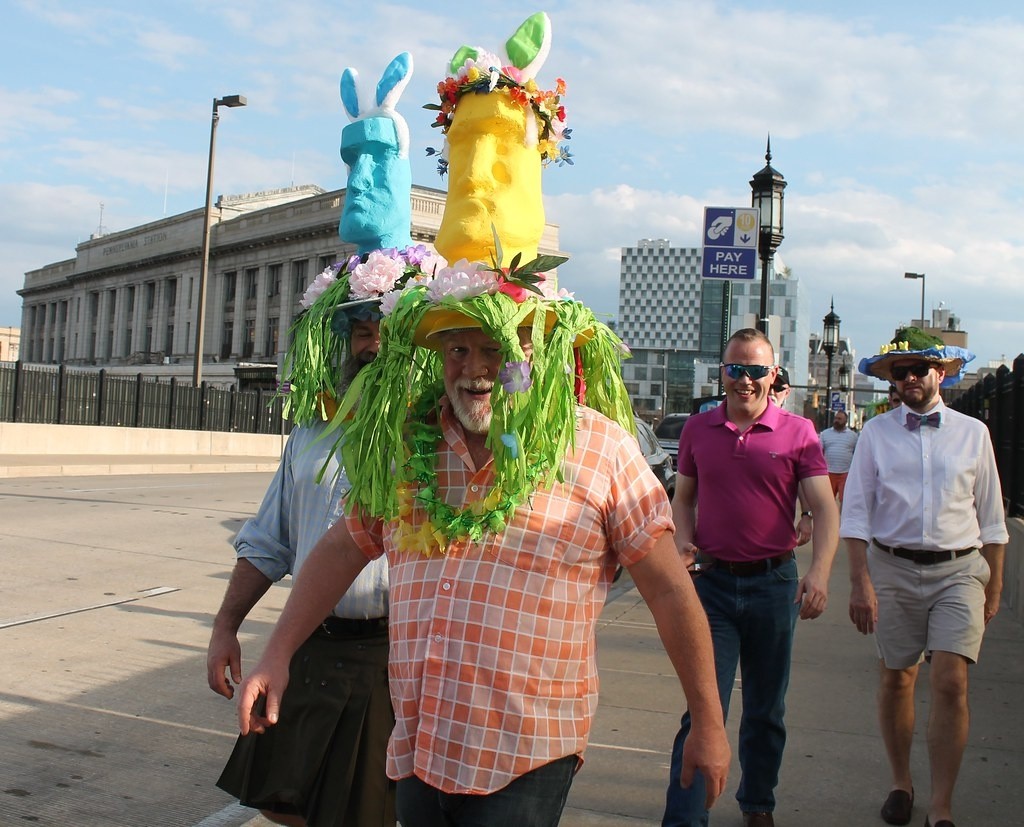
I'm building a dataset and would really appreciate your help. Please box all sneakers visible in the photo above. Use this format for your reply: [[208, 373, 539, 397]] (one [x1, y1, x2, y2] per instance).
[[881, 786, 914, 826], [924, 814, 956, 827]]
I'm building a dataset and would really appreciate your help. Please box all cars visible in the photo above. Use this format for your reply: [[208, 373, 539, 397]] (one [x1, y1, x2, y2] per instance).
[[632, 411, 677, 494], [654, 413, 691, 470]]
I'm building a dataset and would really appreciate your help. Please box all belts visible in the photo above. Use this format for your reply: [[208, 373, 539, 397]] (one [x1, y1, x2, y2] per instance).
[[872, 538, 976, 565], [696, 549, 795, 577]]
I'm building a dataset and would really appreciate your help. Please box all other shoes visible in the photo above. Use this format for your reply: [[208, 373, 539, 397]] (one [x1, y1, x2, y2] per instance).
[[743, 811, 774, 827]]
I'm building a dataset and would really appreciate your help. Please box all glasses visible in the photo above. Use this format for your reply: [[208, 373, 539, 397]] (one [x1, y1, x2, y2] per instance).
[[889, 363, 938, 381], [769, 385, 787, 392], [719, 363, 770, 380]]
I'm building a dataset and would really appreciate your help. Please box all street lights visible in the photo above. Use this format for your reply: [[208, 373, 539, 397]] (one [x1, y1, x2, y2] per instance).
[[811, 333, 819, 429], [748, 132, 788, 338], [904, 272, 925, 330], [193, 94, 248, 389], [823, 296, 841, 428]]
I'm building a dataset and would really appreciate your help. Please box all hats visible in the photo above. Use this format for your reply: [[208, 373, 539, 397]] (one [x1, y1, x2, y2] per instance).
[[777, 366, 790, 386], [858, 326, 977, 387]]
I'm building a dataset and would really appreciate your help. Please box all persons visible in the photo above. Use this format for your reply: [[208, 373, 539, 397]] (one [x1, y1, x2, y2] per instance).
[[658, 328, 840, 827], [237, 254, 733, 827], [206, 242, 448, 827], [841, 326, 1010, 827], [819, 410, 858, 516]]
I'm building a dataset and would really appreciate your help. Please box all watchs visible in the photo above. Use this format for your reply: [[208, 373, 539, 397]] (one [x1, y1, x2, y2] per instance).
[[801, 511, 813, 518]]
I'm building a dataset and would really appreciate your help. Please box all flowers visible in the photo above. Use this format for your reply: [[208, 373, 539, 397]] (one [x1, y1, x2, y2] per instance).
[[299, 241, 586, 318]]
